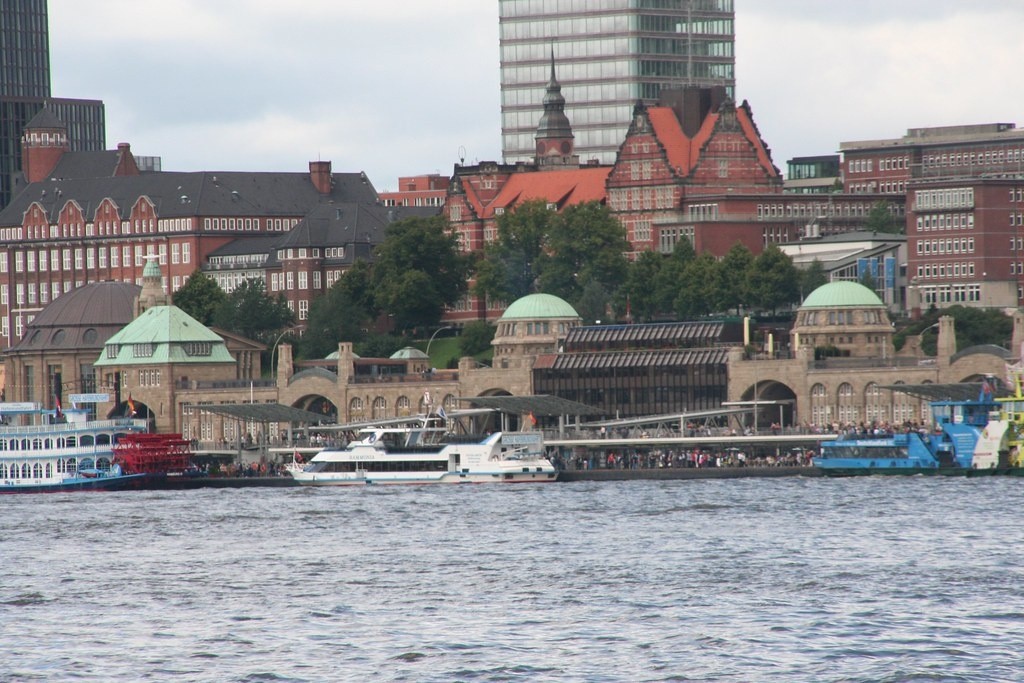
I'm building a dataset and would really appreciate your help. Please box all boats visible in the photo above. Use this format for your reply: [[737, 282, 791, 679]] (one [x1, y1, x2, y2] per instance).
[[0, 389, 204, 491], [811, 371, 1004, 486], [961, 370, 1024, 481], [281, 404, 561, 484]]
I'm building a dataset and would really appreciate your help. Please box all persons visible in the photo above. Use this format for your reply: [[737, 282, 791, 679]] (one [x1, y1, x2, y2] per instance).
[[542, 417, 944, 471], [114, 422, 362, 478]]
